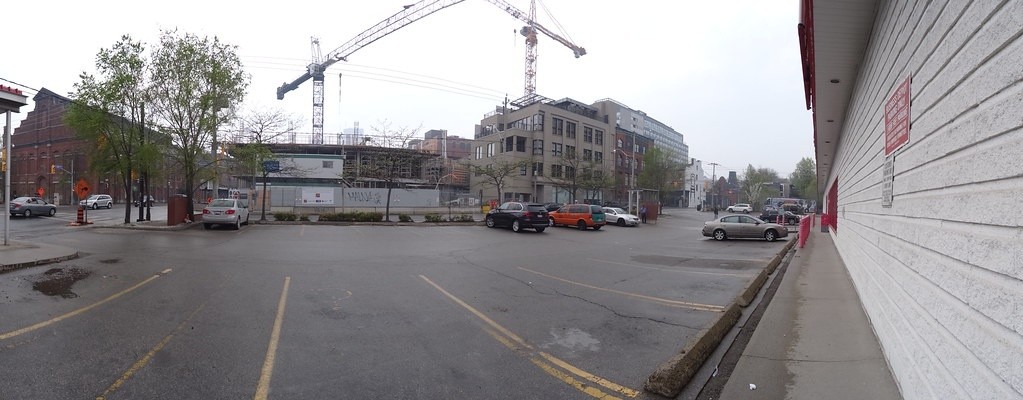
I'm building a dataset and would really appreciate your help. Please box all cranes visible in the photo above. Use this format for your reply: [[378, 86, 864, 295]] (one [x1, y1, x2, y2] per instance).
[[277, 0, 466, 146], [482, 0, 589, 105]]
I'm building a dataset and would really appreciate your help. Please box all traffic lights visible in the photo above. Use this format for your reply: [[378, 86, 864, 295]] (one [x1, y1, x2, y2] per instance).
[[2, 161, 6, 172], [51, 164, 56, 175]]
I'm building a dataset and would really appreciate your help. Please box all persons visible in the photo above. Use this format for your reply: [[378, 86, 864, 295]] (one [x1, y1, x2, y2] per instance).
[[207, 197, 211, 204], [714, 207, 718, 219], [640, 205, 648, 223]]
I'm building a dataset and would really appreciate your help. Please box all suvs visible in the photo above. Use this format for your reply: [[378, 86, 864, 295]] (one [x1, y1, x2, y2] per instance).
[[548, 203, 607, 232], [80, 196, 113, 210], [726, 203, 754, 214], [485, 202, 550, 233]]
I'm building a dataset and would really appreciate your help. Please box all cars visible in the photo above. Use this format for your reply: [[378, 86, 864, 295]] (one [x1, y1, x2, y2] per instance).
[[603, 207, 641, 227], [10, 196, 57, 218], [134, 195, 155, 207], [701, 214, 789, 243], [759, 204, 805, 225], [202, 199, 249, 229]]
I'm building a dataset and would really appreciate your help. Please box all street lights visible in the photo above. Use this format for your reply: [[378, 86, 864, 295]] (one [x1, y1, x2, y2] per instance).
[[611, 149, 635, 190]]
